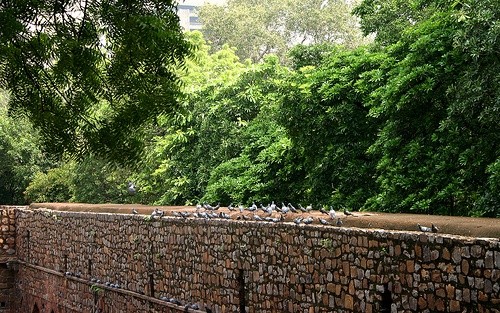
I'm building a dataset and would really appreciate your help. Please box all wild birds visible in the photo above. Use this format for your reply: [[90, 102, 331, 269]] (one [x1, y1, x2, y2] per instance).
[[132, 209, 138, 214], [151, 201, 352, 227], [418, 223, 439, 233]]
